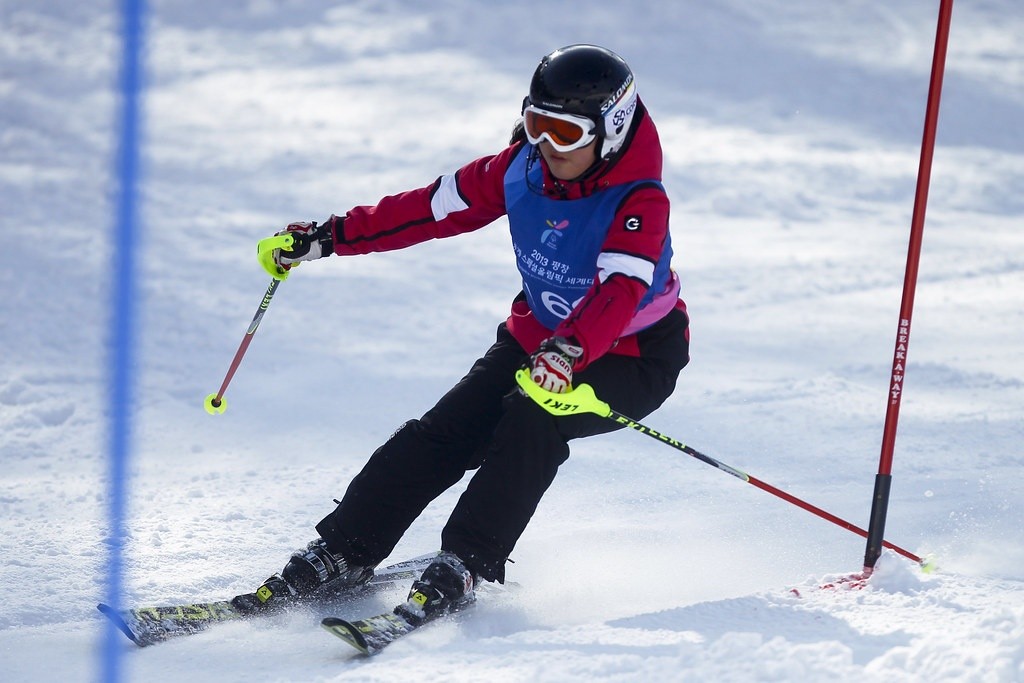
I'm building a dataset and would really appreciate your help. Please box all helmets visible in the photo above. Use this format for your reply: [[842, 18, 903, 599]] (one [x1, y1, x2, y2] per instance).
[[521, 43, 638, 162]]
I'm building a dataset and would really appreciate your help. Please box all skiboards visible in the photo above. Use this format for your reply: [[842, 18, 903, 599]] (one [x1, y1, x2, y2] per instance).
[[319, 577, 523, 660], [96, 547, 443, 651]]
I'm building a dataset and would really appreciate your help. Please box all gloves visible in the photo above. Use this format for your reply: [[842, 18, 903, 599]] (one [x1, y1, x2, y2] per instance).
[[515, 336, 584, 398], [273, 214, 335, 270]]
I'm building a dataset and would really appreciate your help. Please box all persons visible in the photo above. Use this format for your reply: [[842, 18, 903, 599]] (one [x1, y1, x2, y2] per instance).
[[257, 44, 690, 619]]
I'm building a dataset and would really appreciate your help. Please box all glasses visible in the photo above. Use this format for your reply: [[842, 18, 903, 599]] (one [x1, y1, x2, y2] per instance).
[[523, 105, 597, 153]]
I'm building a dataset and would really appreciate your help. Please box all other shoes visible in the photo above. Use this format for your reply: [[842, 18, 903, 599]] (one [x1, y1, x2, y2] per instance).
[[416, 549, 479, 611], [284, 537, 373, 596]]
